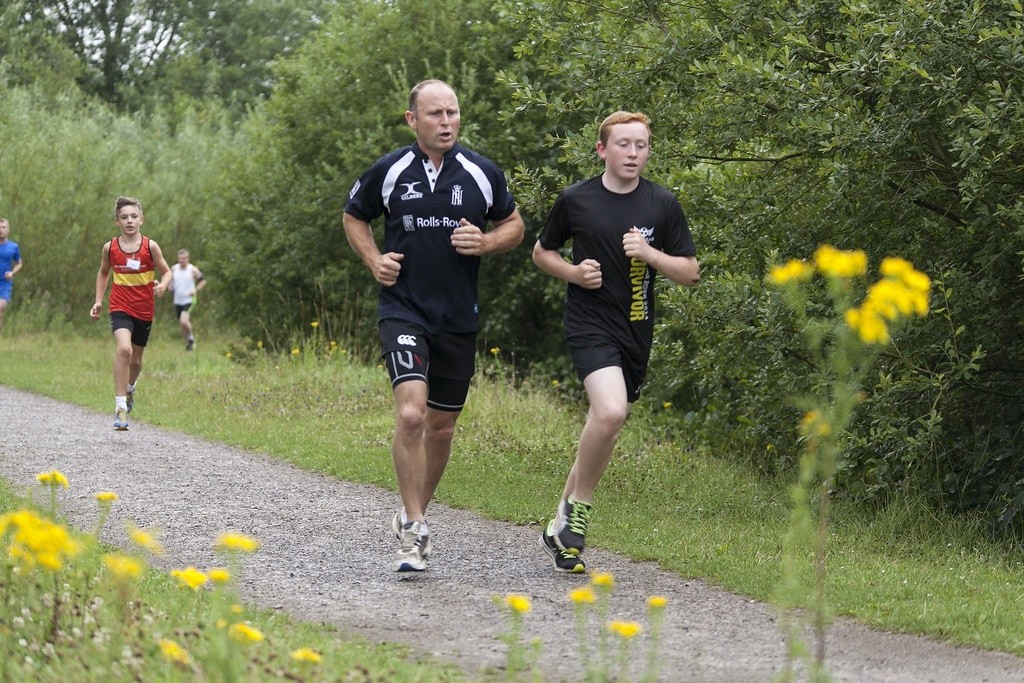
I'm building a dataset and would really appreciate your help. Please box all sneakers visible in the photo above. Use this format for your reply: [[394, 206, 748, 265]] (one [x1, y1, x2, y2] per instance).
[[392, 505, 431, 571], [553, 492, 592, 555], [538, 519, 586, 573], [113, 407, 129, 430], [126, 387, 135, 413]]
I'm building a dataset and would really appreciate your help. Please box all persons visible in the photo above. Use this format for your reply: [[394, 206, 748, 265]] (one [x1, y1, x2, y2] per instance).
[[0, 218, 24, 330], [167, 248, 211, 352], [533, 109, 702, 575], [340, 79, 524, 579], [90, 197, 170, 432]]
